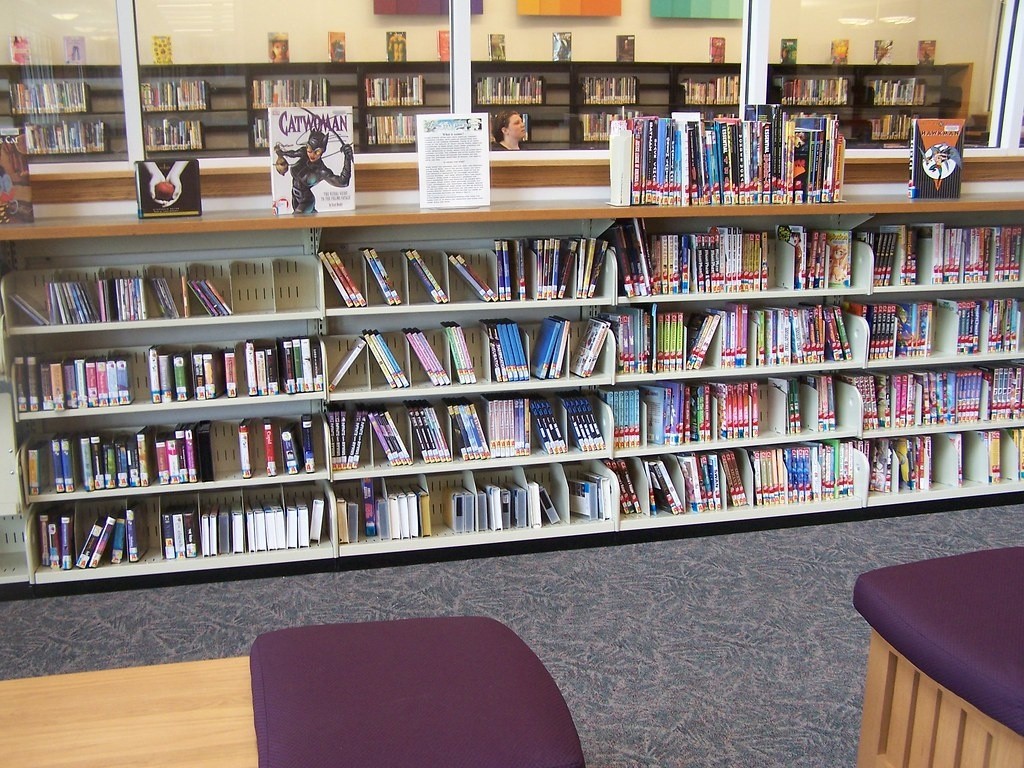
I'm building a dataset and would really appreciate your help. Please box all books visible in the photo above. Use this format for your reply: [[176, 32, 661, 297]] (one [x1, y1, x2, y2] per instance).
[[267, 106, 355, 216], [0, 128, 36, 225], [415, 113, 491, 208], [908, 119, 965, 200], [134, 159, 202, 219], [0, 31, 1023, 570]]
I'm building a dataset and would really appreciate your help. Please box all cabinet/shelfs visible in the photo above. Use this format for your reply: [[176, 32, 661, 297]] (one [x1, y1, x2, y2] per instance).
[[1, 192, 1023, 596], [0, 61, 974, 163]]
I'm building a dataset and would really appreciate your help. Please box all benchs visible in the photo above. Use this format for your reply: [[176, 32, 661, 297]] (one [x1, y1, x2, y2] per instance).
[[0, 616, 587, 768], [851, 548, 1023, 768]]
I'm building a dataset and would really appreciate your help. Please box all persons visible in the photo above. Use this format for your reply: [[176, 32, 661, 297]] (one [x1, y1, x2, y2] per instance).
[[269, 35, 289, 63], [13, 36, 28, 65], [492, 110, 529, 151]]
